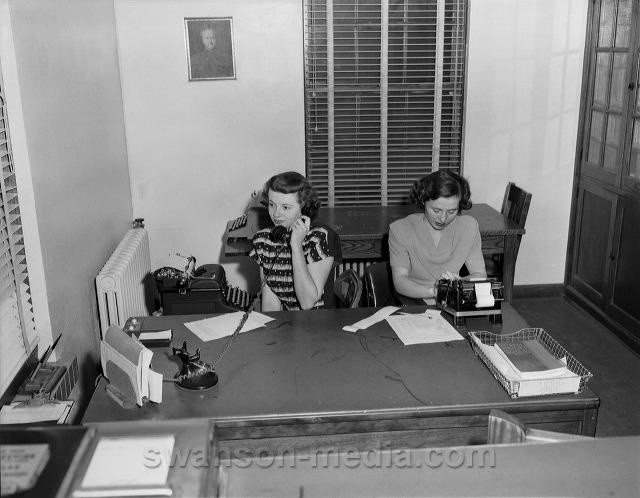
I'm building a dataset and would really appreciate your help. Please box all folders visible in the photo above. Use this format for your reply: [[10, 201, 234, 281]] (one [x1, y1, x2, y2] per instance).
[[0, 386, 75, 426]]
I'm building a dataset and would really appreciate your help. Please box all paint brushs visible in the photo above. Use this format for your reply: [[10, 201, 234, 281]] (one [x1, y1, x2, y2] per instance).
[[30, 345, 50, 382], [42, 333, 63, 367]]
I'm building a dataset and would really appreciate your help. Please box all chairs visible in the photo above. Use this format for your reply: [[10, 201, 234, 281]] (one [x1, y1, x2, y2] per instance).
[[367, 262, 402, 307], [487, 181, 531, 300], [334, 271, 362, 306]]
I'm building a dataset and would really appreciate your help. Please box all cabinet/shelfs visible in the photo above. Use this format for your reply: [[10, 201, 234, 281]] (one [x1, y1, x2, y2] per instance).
[[565, 1, 640, 357]]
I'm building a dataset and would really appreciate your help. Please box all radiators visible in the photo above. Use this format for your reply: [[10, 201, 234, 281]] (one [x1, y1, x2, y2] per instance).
[[95, 223, 151, 342]]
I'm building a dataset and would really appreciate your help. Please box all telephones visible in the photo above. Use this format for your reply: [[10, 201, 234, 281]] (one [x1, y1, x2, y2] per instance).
[[269, 199, 320, 243]]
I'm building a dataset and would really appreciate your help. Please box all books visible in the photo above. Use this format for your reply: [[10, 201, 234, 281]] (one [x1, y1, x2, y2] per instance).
[[492, 340, 569, 375], [0, 444, 51, 498], [57, 422, 220, 498]]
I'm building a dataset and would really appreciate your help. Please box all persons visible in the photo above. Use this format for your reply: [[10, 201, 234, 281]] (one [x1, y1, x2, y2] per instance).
[[191, 28, 233, 78], [389, 171, 487, 305], [248, 172, 341, 310]]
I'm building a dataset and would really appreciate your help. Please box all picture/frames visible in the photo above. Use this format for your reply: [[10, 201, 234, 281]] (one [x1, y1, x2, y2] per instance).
[[182, 16, 236, 81]]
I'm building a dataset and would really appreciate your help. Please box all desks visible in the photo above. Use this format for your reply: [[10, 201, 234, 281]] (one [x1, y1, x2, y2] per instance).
[[84, 309, 602, 463], [313, 201, 526, 306]]
